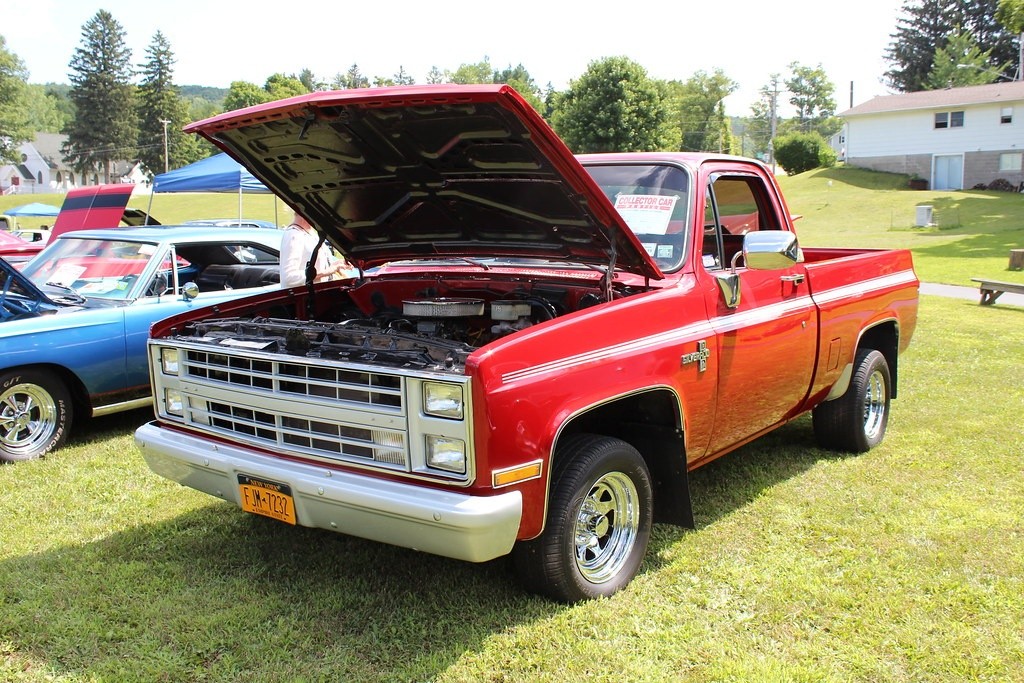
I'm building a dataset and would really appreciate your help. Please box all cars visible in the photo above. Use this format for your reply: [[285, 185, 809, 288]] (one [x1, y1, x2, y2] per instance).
[[0, 223, 349, 463], [0, 182, 191, 285]]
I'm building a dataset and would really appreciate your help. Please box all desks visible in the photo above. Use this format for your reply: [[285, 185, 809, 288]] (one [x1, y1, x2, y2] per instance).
[[11, 229, 50, 240]]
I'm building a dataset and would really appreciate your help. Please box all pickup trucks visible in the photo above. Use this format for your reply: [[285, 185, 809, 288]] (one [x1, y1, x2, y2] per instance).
[[130, 82, 920, 604]]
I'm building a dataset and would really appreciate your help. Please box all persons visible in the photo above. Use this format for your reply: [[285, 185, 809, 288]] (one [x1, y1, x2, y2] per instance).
[[41, 225, 49, 230], [280, 211, 354, 288]]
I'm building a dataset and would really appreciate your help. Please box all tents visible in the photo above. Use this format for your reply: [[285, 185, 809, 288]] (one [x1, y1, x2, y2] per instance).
[[145, 153, 278, 228], [4, 203, 60, 230]]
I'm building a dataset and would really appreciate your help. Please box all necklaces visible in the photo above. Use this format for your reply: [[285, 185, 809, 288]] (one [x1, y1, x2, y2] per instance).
[[288, 223, 310, 235]]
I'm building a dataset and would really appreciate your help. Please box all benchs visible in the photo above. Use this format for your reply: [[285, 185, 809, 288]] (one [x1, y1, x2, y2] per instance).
[[639, 233, 744, 267], [198, 263, 280, 293], [971, 278, 1024, 305]]
